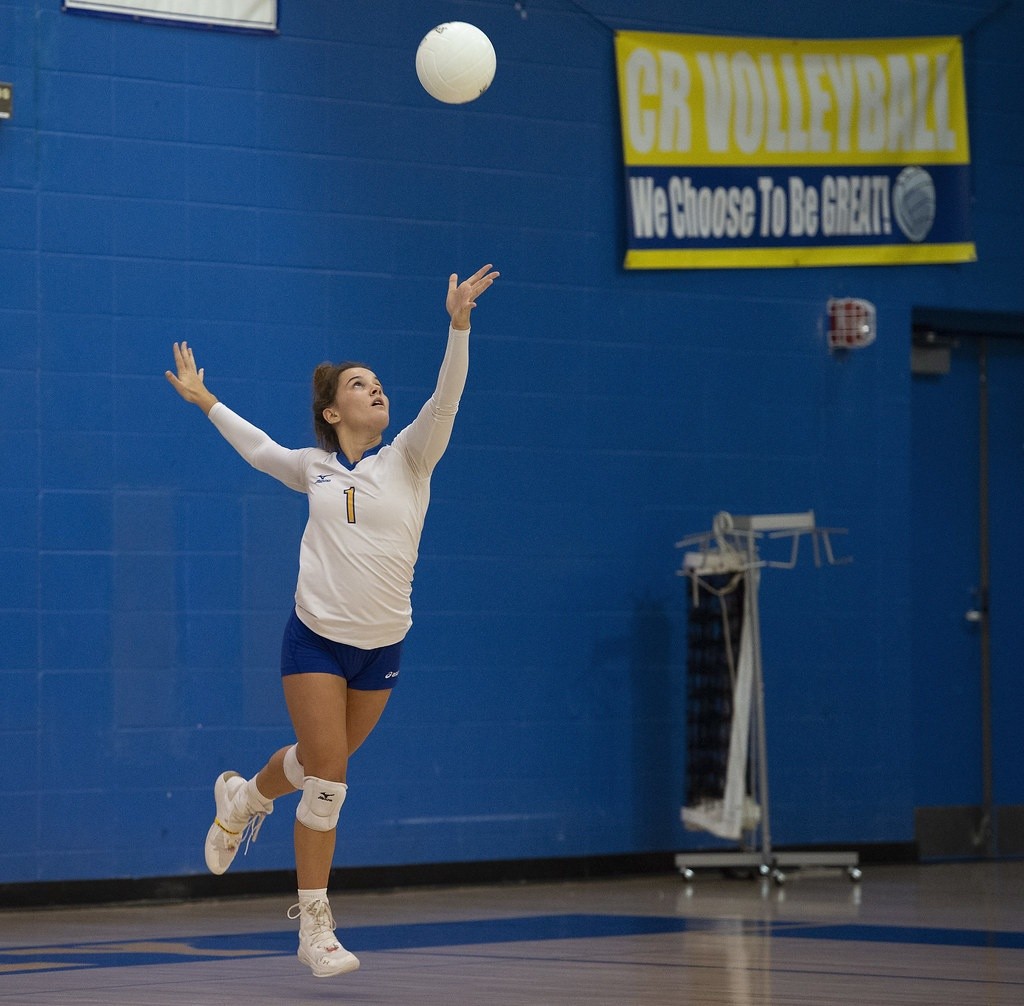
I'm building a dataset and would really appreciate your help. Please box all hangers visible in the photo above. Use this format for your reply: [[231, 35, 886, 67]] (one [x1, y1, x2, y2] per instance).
[[684, 511, 759, 576]]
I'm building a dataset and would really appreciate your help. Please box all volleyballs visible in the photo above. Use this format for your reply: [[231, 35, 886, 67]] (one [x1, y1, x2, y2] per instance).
[[415, 21, 498, 103]]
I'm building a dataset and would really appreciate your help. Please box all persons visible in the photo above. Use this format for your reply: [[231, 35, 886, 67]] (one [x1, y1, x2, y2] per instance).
[[166, 264, 500, 977]]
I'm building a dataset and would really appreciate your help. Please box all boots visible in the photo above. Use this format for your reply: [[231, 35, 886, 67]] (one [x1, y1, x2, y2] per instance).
[[286, 899, 359, 978], [204, 770, 274, 876]]
